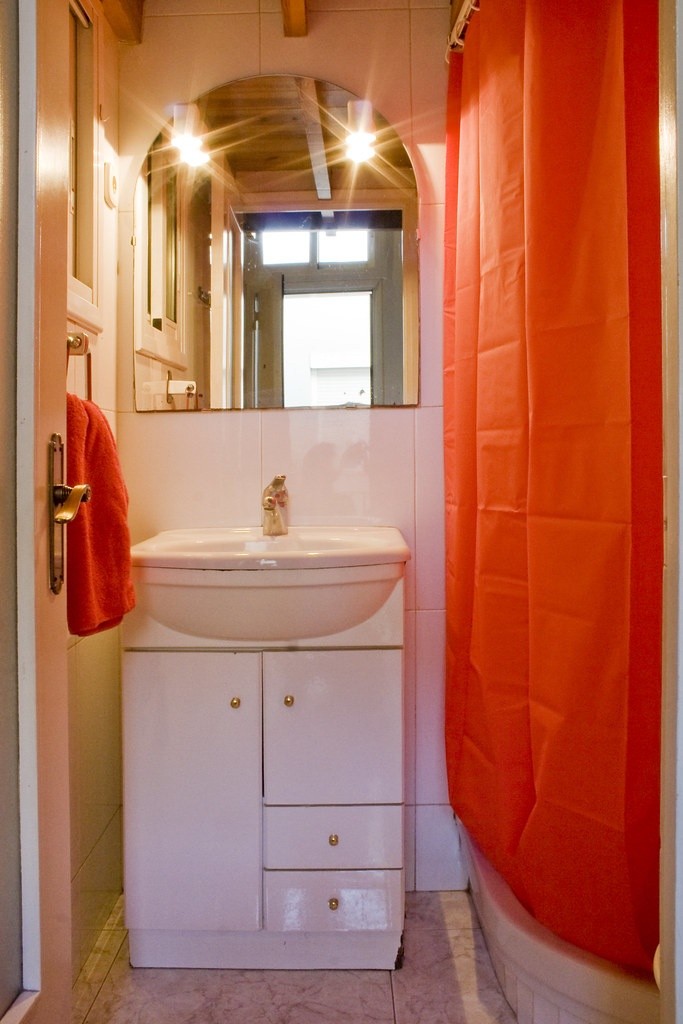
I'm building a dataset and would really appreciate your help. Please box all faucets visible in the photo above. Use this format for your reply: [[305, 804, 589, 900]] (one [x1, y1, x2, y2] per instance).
[[259, 474, 293, 538]]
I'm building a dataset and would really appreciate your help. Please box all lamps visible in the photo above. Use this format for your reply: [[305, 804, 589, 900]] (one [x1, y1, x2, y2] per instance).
[[343, 100, 376, 144], [171, 103, 200, 146], [180, 121, 211, 167], [345, 145, 375, 164]]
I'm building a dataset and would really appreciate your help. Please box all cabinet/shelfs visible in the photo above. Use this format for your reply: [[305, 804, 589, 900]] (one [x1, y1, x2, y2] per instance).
[[118, 576, 409, 971]]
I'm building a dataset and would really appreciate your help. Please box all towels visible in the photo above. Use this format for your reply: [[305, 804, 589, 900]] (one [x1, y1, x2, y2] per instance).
[[65, 390, 137, 637]]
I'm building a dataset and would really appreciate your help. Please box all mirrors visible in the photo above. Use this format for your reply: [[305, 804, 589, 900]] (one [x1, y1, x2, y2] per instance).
[[129, 72, 421, 414]]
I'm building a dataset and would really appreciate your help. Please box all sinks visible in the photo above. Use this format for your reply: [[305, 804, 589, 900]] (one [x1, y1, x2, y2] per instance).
[[129, 527, 412, 641]]
